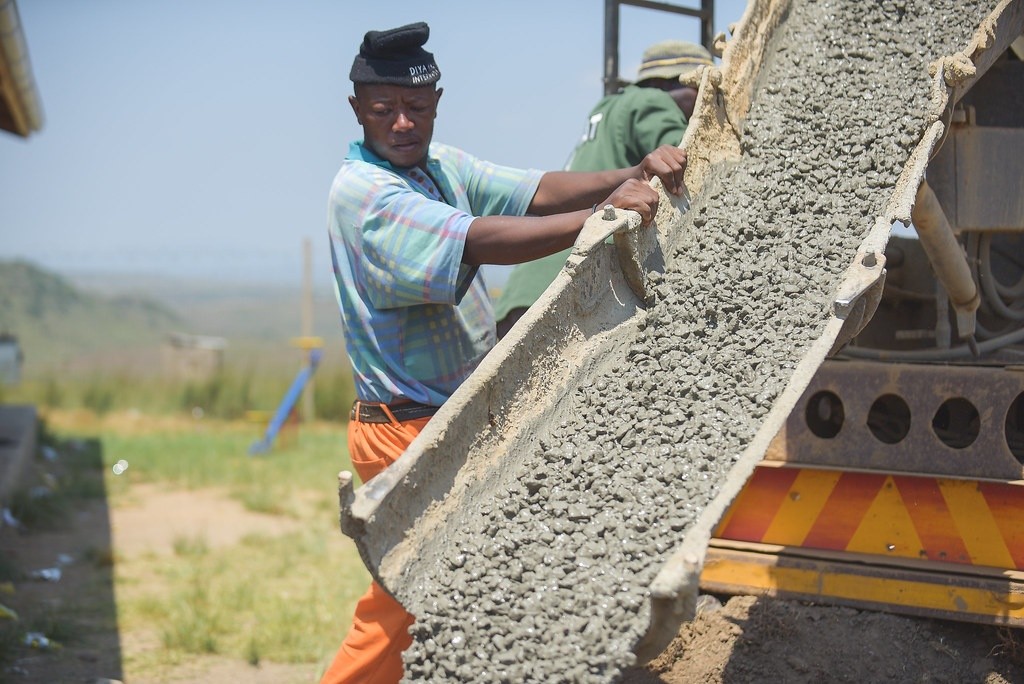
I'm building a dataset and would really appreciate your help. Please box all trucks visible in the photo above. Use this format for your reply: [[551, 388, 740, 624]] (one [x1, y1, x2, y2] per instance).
[[598, 0, 1023, 635]]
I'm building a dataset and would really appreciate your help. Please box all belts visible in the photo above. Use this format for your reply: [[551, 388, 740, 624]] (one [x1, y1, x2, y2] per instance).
[[351, 400, 440, 423]]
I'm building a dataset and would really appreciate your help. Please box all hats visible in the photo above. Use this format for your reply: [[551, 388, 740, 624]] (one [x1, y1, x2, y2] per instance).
[[349, 21, 441, 87], [634, 41, 714, 85]]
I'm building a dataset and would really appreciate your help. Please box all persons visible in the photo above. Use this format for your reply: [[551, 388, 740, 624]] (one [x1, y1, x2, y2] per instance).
[[311, 20, 689, 683], [494, 37, 716, 343]]
